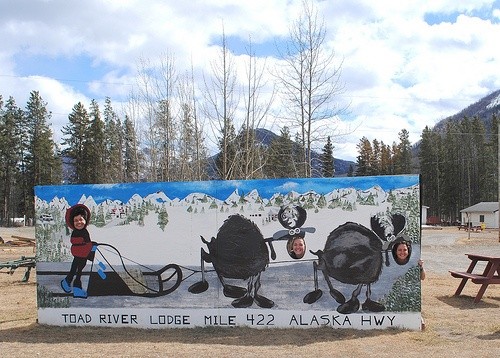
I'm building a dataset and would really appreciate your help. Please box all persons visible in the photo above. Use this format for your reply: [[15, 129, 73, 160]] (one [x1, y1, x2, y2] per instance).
[[72, 210, 87, 229], [289, 237, 306, 259], [392, 241, 426, 280]]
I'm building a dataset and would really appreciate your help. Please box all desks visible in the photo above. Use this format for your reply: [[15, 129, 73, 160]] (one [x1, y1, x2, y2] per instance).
[[454, 253, 500, 302]]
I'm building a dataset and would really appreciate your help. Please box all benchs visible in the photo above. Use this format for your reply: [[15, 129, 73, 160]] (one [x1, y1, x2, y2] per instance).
[[449, 270, 487, 302]]
